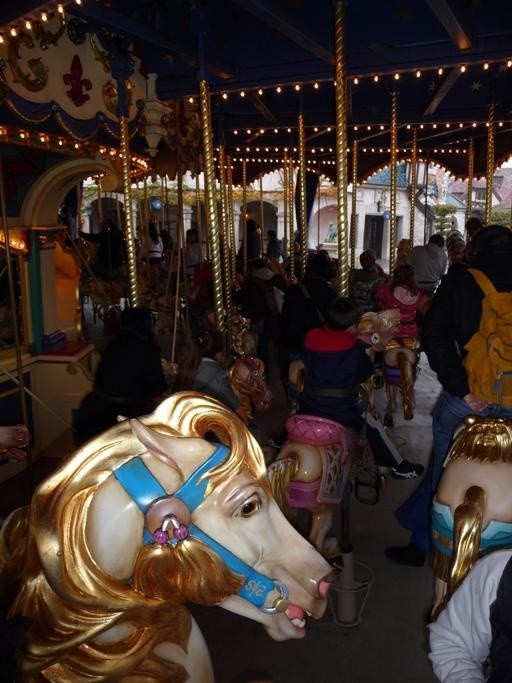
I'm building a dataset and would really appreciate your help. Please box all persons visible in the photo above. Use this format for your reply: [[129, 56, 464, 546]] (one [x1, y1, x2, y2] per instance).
[[81, 219, 199, 285], [188, 218, 282, 337], [284, 248, 425, 480], [78, 310, 167, 437], [384, 218, 511, 566]]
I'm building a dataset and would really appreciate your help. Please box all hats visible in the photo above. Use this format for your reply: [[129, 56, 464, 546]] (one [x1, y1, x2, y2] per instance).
[[322, 294, 359, 331]]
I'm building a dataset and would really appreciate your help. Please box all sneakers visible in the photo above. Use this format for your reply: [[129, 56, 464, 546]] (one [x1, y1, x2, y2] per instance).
[[391, 460, 424, 481], [268, 432, 281, 452]]
